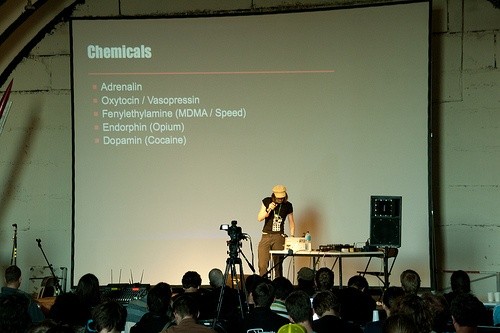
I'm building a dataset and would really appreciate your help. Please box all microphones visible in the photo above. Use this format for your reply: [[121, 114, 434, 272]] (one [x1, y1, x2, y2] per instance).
[[265, 209, 271, 217]]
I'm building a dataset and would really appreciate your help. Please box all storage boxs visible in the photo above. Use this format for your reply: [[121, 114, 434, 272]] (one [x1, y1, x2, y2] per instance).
[[285, 237, 305, 251]]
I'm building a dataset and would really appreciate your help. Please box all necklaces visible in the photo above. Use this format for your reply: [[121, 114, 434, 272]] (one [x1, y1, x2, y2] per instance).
[[274, 203, 281, 220]]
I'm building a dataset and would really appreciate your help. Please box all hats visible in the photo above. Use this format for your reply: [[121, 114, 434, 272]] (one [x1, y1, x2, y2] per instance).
[[272, 184, 287, 199], [278, 323, 308, 333], [295, 267, 314, 280]]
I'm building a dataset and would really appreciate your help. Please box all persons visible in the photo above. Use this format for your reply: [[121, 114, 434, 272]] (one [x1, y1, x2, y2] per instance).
[[130, 268, 494, 333], [258, 184, 295, 281], [0, 265, 43, 333], [42, 273, 128, 333]]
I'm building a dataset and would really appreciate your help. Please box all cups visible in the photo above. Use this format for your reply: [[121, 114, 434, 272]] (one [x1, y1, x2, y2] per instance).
[[488, 292, 493, 302], [495, 292, 500, 302]]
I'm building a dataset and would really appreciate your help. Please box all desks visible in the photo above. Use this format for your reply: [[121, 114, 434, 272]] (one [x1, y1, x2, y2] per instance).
[[269, 249, 390, 297]]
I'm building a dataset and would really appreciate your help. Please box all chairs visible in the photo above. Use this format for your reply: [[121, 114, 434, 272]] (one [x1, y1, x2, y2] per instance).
[[357, 248, 398, 300]]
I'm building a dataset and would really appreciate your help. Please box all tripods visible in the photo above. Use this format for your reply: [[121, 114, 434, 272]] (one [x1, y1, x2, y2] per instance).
[[216, 240, 255, 321]]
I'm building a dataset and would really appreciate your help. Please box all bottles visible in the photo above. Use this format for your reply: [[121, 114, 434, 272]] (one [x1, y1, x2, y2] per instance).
[[305, 231, 312, 251]]
